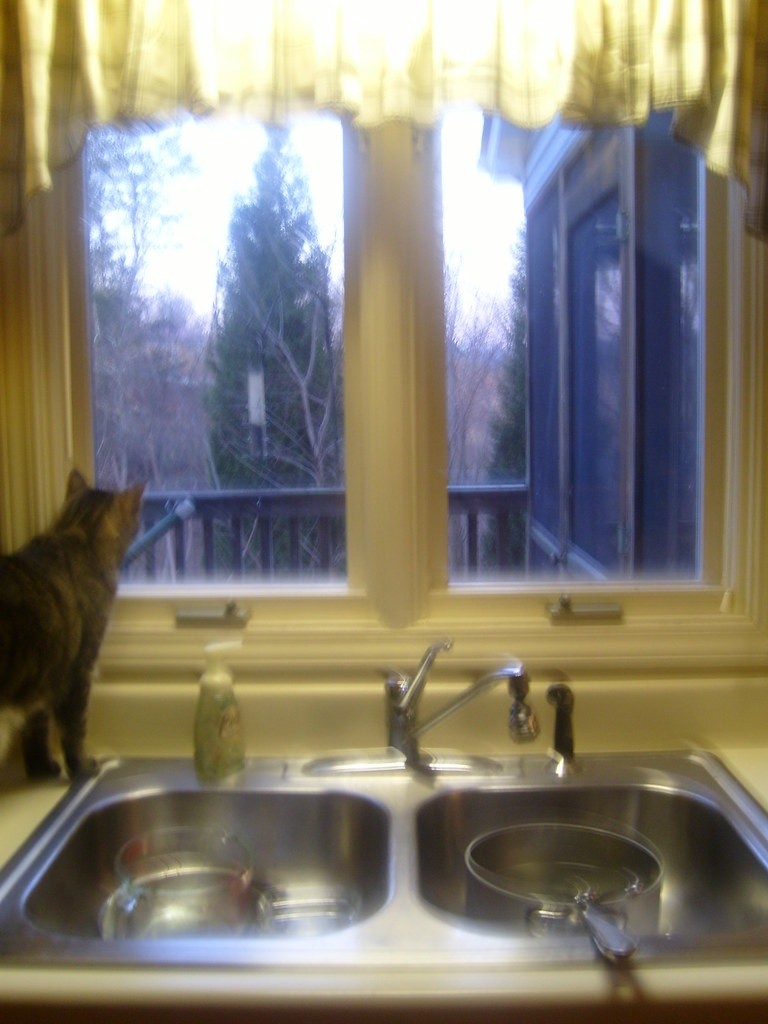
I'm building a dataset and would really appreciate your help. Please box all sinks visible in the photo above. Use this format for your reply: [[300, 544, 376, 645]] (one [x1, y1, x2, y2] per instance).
[[412, 746, 768, 971], [0, 752, 393, 968]]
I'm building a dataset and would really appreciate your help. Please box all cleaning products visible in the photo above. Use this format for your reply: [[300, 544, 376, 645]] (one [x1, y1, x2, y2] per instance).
[[192, 640, 245, 786]]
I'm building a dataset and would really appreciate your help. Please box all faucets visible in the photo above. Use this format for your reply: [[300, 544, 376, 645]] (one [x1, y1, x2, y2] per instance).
[[384, 639, 539, 751]]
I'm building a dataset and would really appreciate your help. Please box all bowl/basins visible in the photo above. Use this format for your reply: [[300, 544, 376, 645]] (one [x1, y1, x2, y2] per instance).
[[466, 822, 668, 965]]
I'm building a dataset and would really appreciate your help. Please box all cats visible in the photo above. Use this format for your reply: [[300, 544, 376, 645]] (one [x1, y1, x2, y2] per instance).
[[0, 470, 149, 780]]
